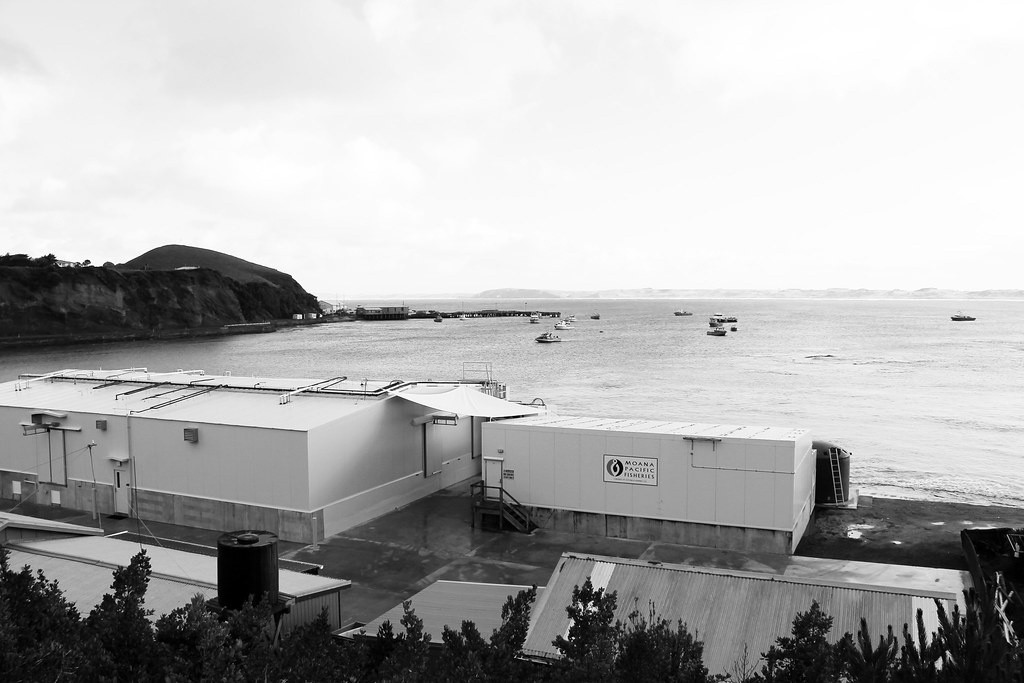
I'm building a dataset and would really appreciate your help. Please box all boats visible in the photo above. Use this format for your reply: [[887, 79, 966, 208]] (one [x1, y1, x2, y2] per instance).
[[707, 327, 727, 336], [591, 314, 600, 319], [565, 315, 577, 321], [674, 312, 692, 316], [731, 327, 737, 331], [554, 320, 575, 330], [530, 312, 540, 323], [708, 315, 737, 327], [951, 314, 976, 321], [434, 313, 442, 322], [535, 332, 561, 342]]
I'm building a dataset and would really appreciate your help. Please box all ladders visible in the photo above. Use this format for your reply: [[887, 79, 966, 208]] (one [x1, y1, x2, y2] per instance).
[[829, 447, 845, 506]]
